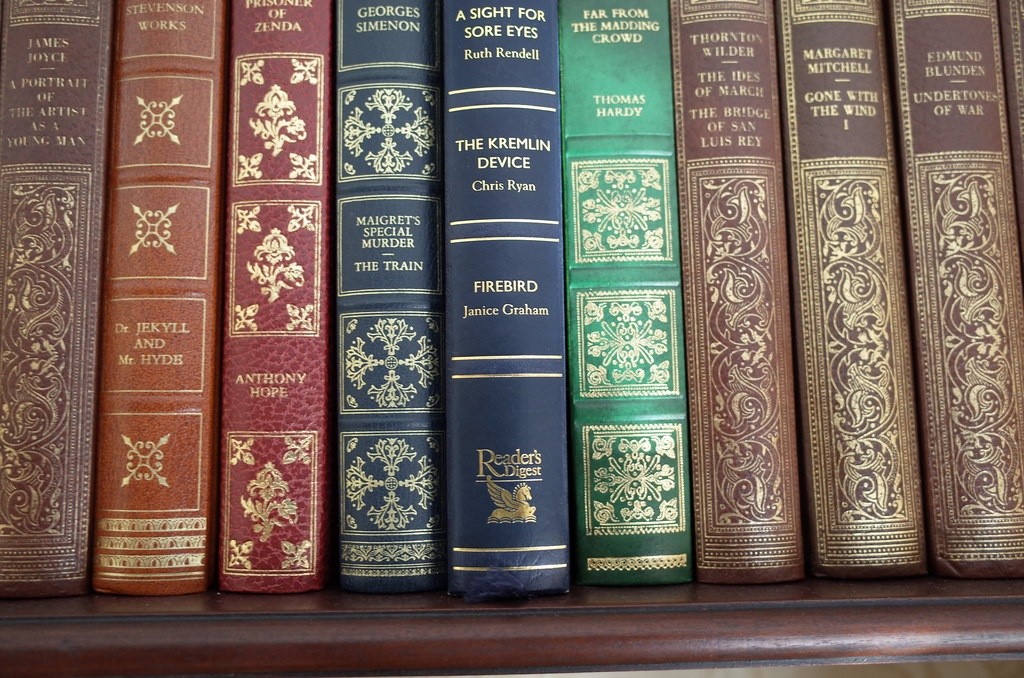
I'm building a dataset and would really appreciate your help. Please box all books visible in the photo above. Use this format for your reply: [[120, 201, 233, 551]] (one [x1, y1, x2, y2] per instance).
[[888, 1, 1022, 579], [219, 0, 333, 594], [668, 0, 803, 580], [89, 0, 226, 599], [999, 2, 1024, 274], [333, 0, 443, 594], [436, 0, 572, 599], [774, 0, 931, 580], [0, 1, 117, 599], [558, 1, 695, 583]]
